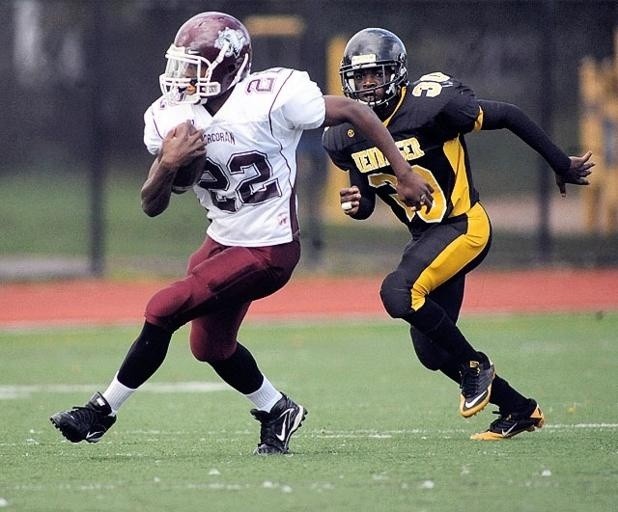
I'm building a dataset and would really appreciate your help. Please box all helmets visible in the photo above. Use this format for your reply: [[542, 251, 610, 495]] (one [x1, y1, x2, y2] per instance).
[[338, 28, 409, 116], [159, 11, 254, 110]]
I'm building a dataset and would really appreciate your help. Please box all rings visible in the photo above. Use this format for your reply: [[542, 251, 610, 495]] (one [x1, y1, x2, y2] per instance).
[[420, 193, 426, 205], [341, 201, 352, 210]]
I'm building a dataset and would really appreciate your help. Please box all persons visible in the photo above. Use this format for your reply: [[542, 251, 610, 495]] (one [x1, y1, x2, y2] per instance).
[[50, 9, 436, 456], [321, 28, 596, 441]]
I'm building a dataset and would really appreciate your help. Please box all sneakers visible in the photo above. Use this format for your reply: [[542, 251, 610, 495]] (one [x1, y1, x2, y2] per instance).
[[458, 350, 496, 418], [470, 398, 545, 441], [48, 390, 119, 445], [251, 390, 308, 457]]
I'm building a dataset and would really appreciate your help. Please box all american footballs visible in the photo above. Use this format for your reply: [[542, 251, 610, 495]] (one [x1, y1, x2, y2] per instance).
[[158, 122, 205, 195]]
